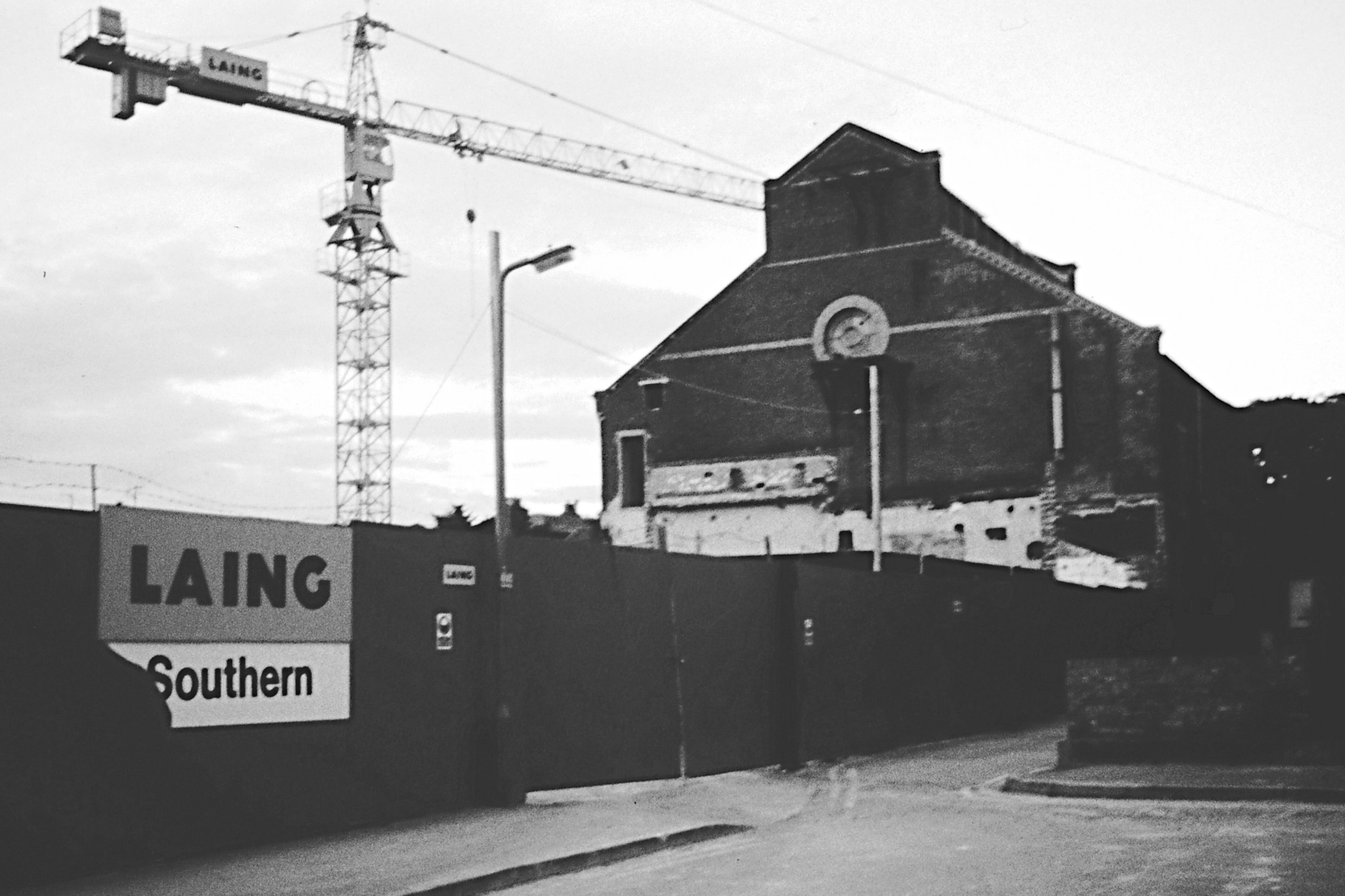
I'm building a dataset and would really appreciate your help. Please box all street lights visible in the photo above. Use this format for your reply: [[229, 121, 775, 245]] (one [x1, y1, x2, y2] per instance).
[[481, 228, 577, 811]]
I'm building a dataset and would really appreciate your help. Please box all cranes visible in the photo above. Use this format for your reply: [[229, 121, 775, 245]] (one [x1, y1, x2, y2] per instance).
[[55, 10, 772, 521]]
